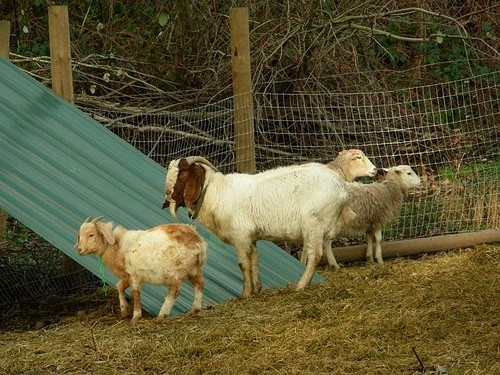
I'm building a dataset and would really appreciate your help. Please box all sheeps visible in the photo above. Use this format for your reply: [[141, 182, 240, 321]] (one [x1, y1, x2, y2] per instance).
[[323, 163, 423, 272], [284, 147, 379, 266], [73, 214, 210, 323], [161, 154, 360, 303]]
